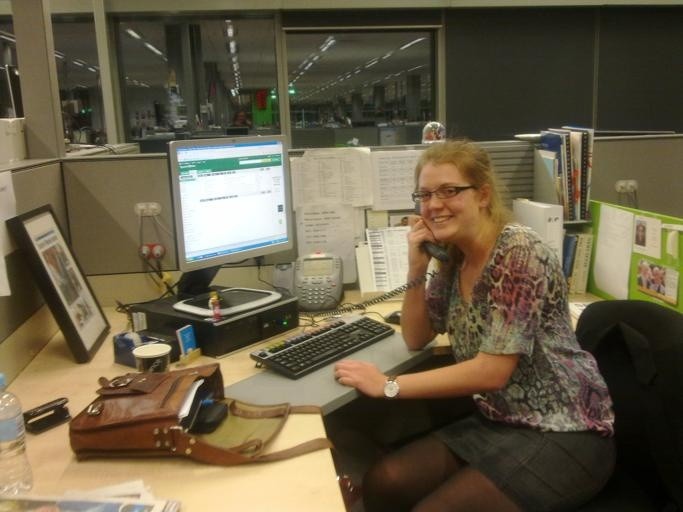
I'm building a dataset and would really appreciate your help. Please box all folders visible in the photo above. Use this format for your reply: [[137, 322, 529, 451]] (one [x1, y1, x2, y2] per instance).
[[512, 197, 564, 273]]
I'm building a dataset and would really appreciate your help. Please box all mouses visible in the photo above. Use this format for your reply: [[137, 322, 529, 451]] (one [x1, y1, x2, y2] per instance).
[[384, 312, 402, 324]]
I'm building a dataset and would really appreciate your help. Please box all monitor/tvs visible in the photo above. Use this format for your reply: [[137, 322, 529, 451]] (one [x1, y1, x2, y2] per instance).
[[164, 135, 296, 318]]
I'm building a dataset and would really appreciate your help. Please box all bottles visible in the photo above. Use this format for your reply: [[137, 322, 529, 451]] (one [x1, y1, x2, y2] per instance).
[[0, 373, 33, 497]]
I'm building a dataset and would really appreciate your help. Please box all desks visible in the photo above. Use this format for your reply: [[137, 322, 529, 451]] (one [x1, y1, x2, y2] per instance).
[[0, 290, 611, 511]]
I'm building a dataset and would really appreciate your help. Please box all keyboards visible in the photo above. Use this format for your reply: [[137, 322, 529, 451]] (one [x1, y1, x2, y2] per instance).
[[251, 313, 395, 380]]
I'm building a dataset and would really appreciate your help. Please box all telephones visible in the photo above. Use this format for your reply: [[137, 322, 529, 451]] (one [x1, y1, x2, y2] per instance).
[[415, 197, 450, 261]]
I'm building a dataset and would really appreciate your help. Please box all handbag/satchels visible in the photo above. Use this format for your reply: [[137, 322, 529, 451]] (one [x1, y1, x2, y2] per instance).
[[68, 362, 341, 467]]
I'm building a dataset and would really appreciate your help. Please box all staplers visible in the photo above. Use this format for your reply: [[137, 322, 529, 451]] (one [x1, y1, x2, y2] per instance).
[[22, 397, 71, 435]]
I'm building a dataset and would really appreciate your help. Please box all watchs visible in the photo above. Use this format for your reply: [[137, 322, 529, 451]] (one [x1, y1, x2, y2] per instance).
[[382, 375, 399, 398]]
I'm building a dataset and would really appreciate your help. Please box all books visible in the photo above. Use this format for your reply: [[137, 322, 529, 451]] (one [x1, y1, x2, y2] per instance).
[[532, 126, 596, 293]]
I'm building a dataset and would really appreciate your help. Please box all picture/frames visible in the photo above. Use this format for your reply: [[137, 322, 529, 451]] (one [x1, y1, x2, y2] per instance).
[[5, 204, 111, 363]]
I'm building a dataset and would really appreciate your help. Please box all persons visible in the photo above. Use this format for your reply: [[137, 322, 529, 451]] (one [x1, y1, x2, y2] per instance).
[[636, 261, 651, 289], [334, 140, 618, 511], [227, 111, 253, 136], [649, 267, 666, 296], [636, 225, 645, 247]]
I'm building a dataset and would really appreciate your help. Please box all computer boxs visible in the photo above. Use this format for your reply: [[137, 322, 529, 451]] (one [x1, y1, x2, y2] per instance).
[[128, 285, 298, 357]]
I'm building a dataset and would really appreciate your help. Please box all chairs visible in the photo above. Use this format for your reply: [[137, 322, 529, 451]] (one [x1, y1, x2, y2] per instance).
[[575, 299, 683, 511]]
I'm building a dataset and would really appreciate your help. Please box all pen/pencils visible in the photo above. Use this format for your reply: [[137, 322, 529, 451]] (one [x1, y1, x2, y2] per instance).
[[201, 399, 220, 406]]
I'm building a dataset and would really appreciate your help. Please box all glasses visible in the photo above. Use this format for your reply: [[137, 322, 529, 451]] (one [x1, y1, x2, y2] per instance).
[[411, 184, 474, 203]]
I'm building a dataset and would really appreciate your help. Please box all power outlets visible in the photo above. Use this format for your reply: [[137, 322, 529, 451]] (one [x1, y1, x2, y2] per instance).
[[133, 202, 162, 217]]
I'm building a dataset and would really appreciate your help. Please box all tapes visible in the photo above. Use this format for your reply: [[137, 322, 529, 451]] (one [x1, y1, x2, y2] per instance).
[[123, 332, 143, 348]]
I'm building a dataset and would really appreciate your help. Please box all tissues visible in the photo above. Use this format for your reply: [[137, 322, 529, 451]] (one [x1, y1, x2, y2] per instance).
[[113, 327, 180, 369]]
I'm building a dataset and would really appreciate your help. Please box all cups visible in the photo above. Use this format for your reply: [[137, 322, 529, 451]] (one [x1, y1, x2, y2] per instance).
[[132, 343, 172, 373]]
